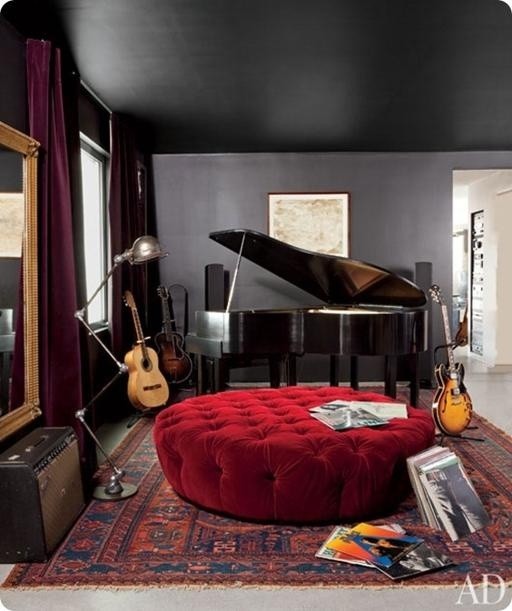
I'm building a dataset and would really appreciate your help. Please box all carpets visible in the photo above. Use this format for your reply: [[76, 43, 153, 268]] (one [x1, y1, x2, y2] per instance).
[[3, 386, 512, 590]]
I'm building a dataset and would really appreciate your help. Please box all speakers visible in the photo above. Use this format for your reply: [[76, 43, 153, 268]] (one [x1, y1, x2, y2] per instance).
[[203, 264, 226, 313], [0, 425, 86, 563], [414, 261, 433, 310]]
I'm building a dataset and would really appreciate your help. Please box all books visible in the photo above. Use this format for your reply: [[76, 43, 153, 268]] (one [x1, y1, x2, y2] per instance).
[[308, 399, 491, 580]]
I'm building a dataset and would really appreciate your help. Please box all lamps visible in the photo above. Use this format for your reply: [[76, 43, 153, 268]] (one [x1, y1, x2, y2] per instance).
[[72, 235, 170, 501]]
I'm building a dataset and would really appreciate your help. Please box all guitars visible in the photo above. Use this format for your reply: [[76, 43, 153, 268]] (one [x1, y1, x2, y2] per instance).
[[123, 290, 169, 411], [154, 285, 193, 384], [428, 285, 473, 436]]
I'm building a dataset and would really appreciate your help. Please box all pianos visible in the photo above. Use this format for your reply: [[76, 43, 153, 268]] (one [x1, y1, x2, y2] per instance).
[[186, 229, 428, 406]]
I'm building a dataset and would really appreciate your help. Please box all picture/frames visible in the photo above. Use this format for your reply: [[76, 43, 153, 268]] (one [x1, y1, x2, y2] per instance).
[[268, 193, 351, 259]]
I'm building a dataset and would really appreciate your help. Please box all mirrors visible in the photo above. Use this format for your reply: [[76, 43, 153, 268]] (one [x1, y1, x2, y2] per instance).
[[0, 122, 42, 443]]
